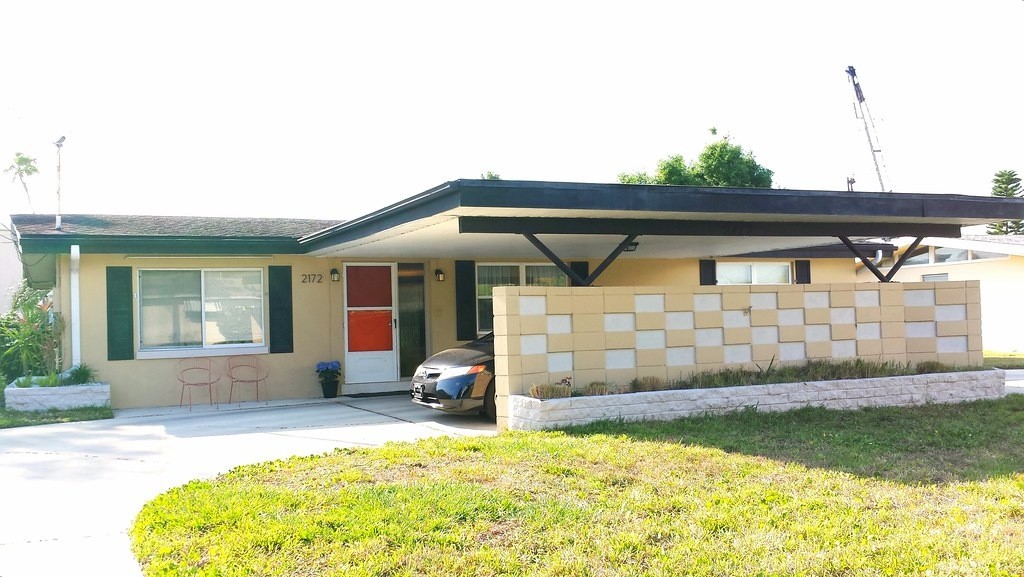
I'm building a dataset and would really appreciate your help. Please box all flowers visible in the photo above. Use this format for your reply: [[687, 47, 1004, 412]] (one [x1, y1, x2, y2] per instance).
[[315, 360, 341, 383]]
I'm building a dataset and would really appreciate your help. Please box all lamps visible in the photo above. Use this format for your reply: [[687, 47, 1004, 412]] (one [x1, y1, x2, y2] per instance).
[[330, 268, 340, 281], [624, 242, 639, 252], [434, 269, 445, 281]]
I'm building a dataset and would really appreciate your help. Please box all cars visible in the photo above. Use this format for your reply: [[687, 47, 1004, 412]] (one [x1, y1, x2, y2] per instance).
[[409, 331, 497, 422]]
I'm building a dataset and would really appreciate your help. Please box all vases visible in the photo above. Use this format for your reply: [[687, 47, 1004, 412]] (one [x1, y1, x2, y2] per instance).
[[321, 380, 339, 398]]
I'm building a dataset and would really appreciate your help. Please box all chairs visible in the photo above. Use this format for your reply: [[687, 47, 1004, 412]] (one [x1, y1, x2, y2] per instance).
[[174, 357, 222, 413], [223, 354, 271, 408]]
